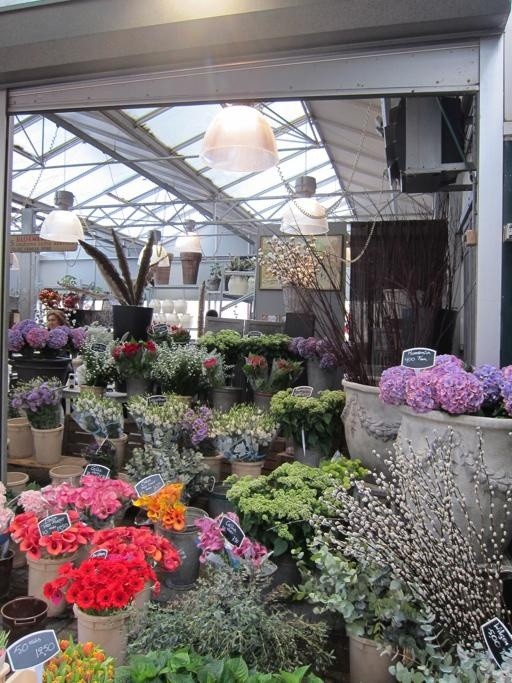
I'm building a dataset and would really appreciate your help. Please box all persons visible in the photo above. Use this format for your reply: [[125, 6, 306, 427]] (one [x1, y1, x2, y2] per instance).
[[45, 311, 66, 329], [206, 308, 218, 317]]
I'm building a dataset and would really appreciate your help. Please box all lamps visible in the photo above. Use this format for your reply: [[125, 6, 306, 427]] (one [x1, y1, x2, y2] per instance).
[[136, 230, 171, 269], [201, 103, 279, 171], [279, 118, 330, 236], [38, 130, 86, 242], [173, 218, 205, 253]]
